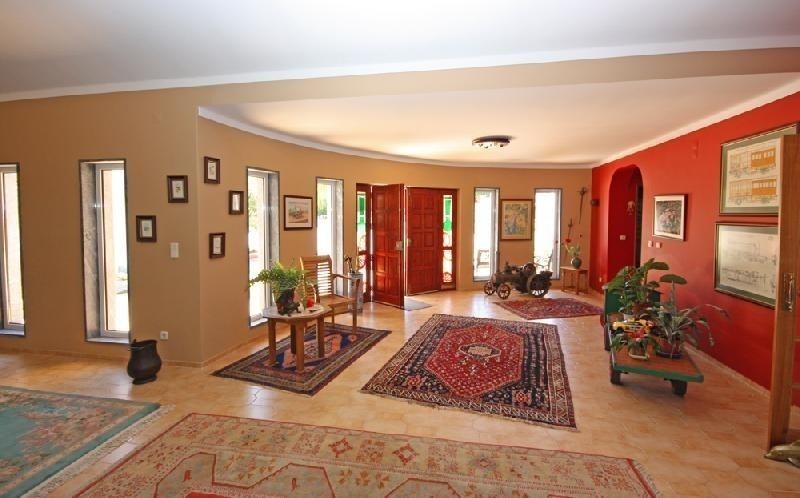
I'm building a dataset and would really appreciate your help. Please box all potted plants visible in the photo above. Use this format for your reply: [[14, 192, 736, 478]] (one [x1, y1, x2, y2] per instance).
[[244, 261, 321, 314], [599, 258, 733, 362]]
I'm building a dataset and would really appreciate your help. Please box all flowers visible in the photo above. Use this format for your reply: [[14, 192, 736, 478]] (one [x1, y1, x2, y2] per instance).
[[344, 254, 363, 273], [559, 232, 584, 258]]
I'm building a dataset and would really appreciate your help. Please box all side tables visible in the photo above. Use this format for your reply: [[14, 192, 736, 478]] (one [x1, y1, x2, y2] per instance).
[[260, 303, 333, 376]]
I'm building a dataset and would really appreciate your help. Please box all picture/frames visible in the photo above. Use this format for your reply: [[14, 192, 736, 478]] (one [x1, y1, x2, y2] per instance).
[[712, 123, 799, 309], [134, 156, 246, 261], [651, 193, 689, 242], [282, 194, 314, 232], [498, 197, 533, 243]]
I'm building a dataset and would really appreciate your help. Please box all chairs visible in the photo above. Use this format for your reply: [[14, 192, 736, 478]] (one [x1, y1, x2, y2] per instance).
[[298, 252, 360, 339]]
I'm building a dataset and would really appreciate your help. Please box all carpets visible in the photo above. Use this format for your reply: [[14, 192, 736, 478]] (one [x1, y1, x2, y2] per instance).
[[358, 297, 605, 433], [209, 320, 393, 400]]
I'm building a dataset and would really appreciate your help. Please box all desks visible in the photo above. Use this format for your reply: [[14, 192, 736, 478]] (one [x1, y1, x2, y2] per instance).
[[559, 264, 590, 295], [603, 324, 704, 397]]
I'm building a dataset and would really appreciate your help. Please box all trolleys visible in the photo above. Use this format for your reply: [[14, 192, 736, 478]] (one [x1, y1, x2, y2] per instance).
[[600, 279, 705, 395]]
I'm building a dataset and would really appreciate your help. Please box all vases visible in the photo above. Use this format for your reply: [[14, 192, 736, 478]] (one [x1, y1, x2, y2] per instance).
[[347, 270, 365, 313], [570, 255, 582, 267]]
